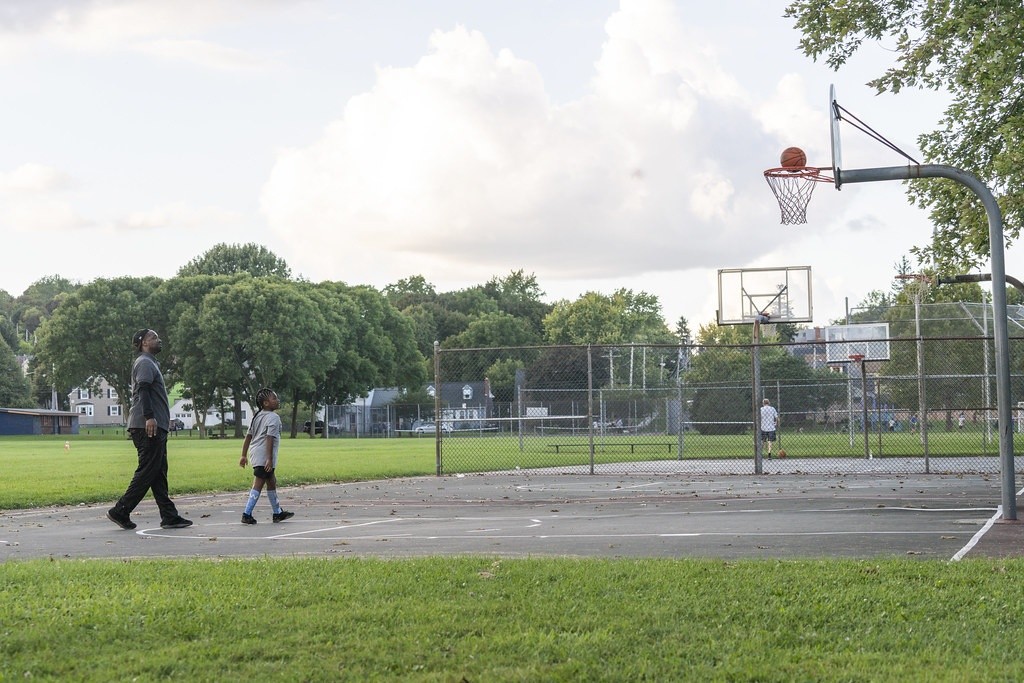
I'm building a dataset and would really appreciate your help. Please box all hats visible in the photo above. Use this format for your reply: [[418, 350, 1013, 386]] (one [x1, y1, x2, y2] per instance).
[[132, 329, 148, 354]]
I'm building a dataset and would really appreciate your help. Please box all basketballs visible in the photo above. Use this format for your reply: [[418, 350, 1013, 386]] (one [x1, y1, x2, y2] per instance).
[[780, 147, 806, 173], [778, 450, 786, 458]]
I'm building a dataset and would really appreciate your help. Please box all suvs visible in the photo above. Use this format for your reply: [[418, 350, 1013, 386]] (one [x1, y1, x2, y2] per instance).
[[169, 418, 184, 431], [304, 420, 339, 435]]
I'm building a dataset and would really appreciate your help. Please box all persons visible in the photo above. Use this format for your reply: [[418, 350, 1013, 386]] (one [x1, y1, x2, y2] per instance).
[[239, 388, 295, 524], [761, 399, 780, 460], [889, 418, 896, 432], [957, 415, 965, 434], [106, 327, 193, 530], [909, 413, 919, 434]]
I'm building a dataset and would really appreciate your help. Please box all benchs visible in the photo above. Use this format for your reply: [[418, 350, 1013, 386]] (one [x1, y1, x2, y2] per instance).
[[211, 433, 227, 438]]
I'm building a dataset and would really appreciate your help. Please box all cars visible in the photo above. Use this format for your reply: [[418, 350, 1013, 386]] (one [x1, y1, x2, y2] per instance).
[[414, 422, 453, 435], [369, 422, 391, 433]]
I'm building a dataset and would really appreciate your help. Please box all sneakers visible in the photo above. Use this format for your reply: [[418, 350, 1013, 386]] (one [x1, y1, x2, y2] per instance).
[[106, 506, 137, 530], [768, 453, 772, 460], [241, 512, 257, 525], [272, 508, 295, 523], [160, 516, 193, 528]]
